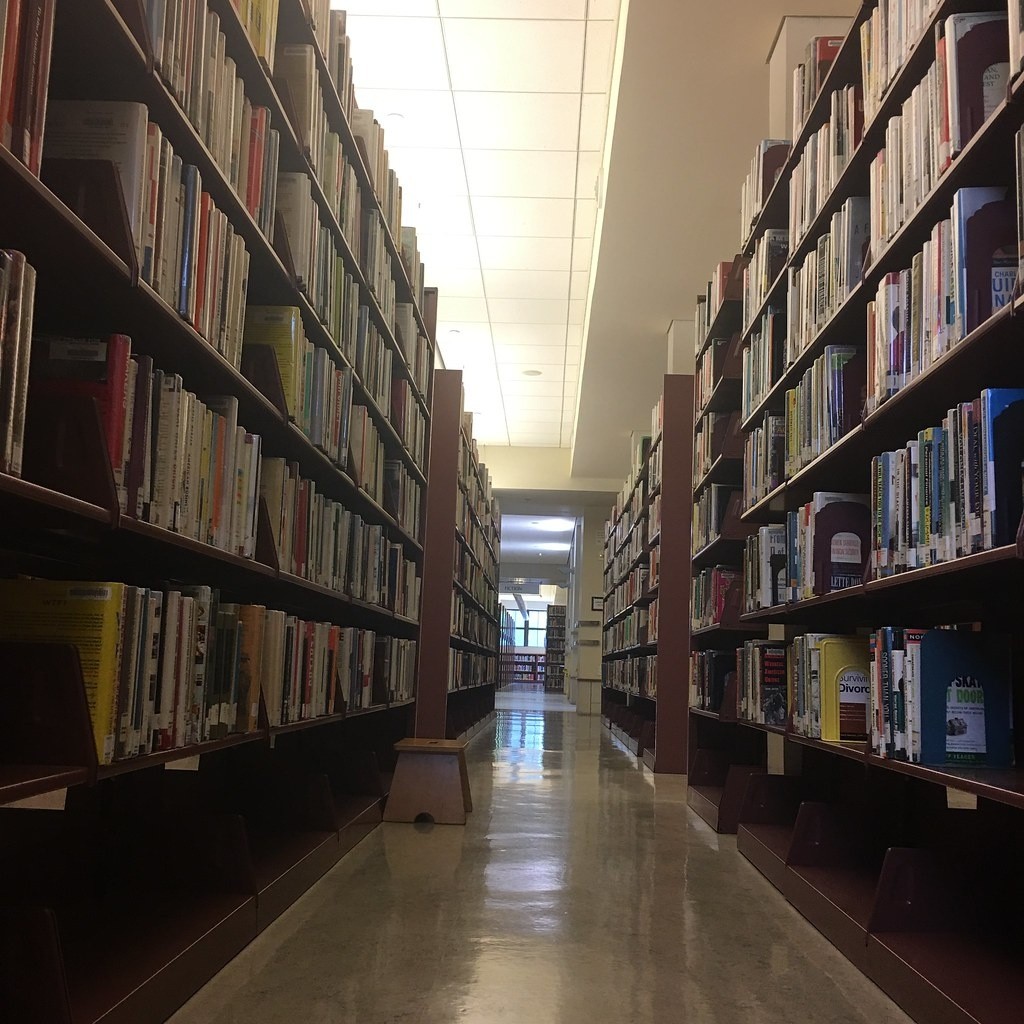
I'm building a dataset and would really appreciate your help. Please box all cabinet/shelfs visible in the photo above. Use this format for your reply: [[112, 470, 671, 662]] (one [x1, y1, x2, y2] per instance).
[[0, 0, 1024, 1024]]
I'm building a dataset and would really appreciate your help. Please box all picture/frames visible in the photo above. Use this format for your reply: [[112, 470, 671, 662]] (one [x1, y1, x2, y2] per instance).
[[591, 597, 603, 612]]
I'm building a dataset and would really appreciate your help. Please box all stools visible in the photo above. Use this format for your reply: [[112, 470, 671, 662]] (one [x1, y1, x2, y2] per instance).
[[382, 736, 472, 826]]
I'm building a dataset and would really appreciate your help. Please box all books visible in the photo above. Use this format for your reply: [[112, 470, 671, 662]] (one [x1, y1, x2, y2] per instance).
[[514, 607, 565, 687], [0, 0, 501, 764], [602, 0, 1024, 767]]
